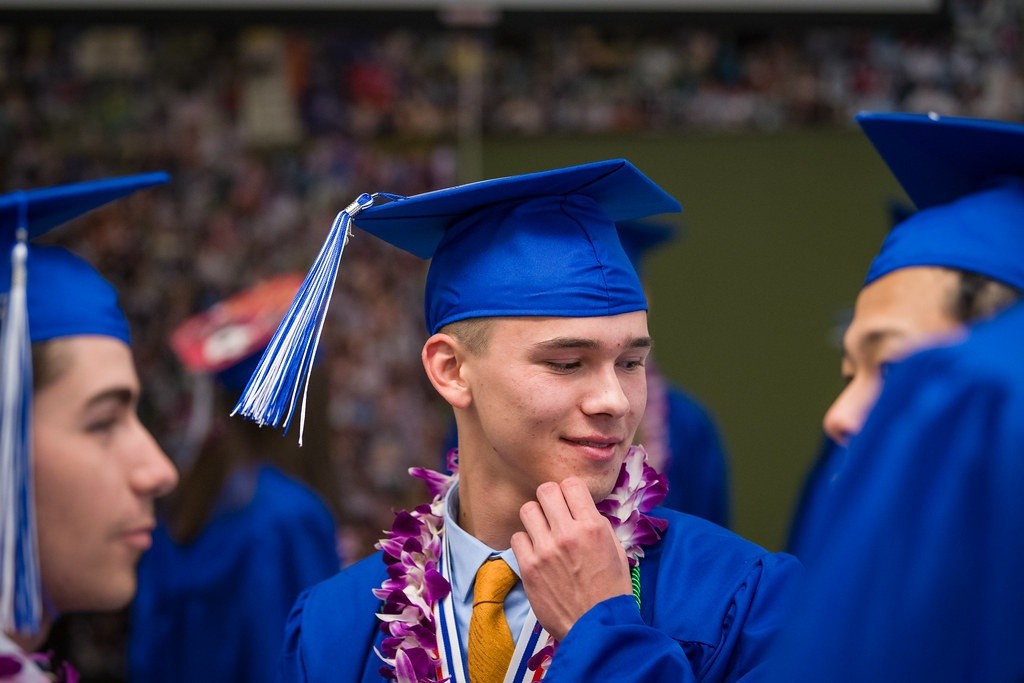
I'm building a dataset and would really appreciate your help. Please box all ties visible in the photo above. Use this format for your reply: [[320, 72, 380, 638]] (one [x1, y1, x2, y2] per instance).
[[465, 557, 525, 681]]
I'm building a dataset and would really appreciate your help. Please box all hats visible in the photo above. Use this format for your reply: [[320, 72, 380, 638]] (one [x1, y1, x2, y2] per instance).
[[2, 171, 178, 633], [228, 159, 686, 454], [853, 111, 1022, 288]]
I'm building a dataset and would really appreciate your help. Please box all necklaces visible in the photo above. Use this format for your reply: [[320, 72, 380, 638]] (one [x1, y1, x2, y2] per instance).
[[372, 444, 670, 683]]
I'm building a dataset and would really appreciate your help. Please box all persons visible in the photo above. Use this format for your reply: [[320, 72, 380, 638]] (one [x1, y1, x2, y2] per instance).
[[271, 159, 797, 683], [740, 114, 1024, 683], [0, 173, 342, 683]]
